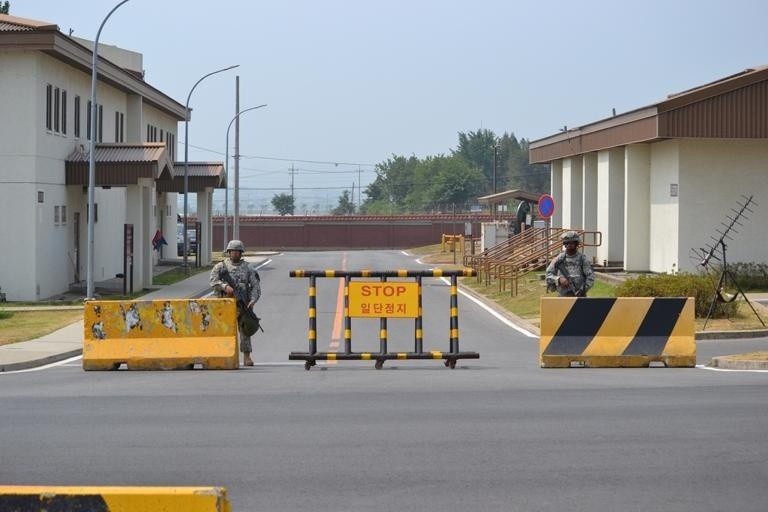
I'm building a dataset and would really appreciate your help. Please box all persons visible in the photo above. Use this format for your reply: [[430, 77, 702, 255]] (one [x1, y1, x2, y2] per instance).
[[544, 230, 596, 298], [210, 238, 261, 366]]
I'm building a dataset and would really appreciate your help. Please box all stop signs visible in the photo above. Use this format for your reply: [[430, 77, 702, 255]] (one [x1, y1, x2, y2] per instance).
[[348, 282, 419, 319]]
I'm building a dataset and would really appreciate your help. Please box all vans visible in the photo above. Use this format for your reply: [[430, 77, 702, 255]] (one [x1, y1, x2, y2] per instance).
[[176, 221, 190, 256], [187, 229, 197, 253]]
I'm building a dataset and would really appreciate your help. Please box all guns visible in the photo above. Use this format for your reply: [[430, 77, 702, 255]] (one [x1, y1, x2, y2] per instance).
[[218, 267, 264, 332], [554, 259, 579, 293]]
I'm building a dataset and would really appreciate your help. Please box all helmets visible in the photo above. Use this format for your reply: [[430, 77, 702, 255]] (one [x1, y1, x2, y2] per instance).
[[226, 240, 246, 252], [560, 232, 581, 244]]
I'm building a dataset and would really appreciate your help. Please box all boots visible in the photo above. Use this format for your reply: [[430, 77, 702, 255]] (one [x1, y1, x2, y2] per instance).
[[244, 352, 254, 365]]
